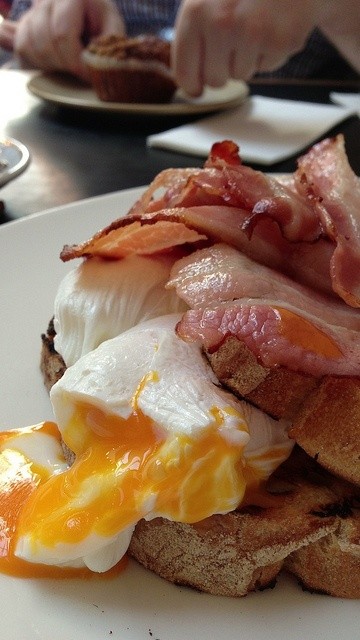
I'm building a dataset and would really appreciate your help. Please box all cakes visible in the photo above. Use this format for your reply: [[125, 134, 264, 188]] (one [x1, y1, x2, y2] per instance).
[[78, 32, 179, 105]]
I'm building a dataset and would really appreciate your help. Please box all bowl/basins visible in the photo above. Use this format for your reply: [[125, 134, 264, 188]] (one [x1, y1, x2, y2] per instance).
[[0, 133, 31, 188]]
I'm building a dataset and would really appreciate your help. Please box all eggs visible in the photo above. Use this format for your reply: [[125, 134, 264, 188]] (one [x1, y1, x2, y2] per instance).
[[0, 310, 294, 582], [48, 249, 181, 371]]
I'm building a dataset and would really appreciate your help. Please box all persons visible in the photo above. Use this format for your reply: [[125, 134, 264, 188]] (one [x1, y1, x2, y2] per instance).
[[0, 0, 41, 49], [16, 1, 360, 98]]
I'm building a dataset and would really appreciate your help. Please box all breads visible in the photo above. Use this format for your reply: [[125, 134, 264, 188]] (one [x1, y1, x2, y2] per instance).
[[195, 321, 360, 485], [129, 468, 360, 607]]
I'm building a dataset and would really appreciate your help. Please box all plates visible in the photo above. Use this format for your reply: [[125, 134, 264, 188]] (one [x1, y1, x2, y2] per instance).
[[26, 71, 249, 116], [0, 171, 360, 638]]
